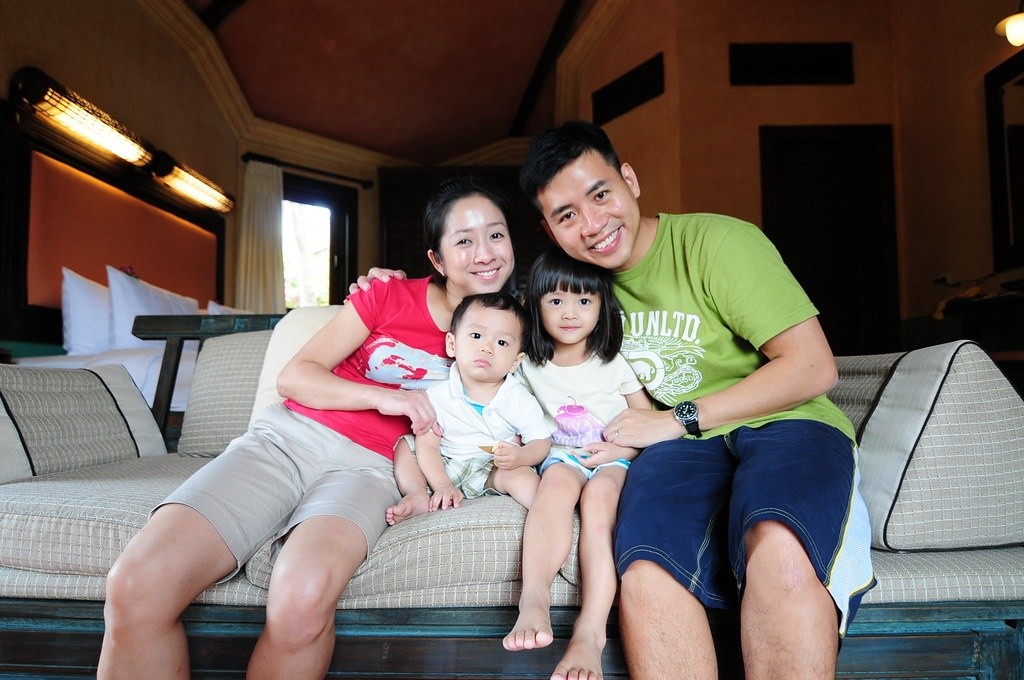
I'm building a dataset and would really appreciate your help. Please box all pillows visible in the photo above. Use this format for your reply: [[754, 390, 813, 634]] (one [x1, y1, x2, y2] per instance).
[[64, 265, 255, 354], [827, 340, 1024, 551]]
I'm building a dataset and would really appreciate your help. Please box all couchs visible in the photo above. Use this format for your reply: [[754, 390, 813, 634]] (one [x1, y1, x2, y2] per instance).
[[0, 307, 1024, 680]]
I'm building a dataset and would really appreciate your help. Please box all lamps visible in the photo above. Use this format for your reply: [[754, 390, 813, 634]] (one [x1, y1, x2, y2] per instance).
[[994, 0, 1024, 47], [16, 67, 152, 164], [152, 147, 235, 212]]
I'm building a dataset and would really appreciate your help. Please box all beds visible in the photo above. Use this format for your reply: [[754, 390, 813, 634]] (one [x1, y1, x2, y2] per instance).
[[0, 98, 256, 443]]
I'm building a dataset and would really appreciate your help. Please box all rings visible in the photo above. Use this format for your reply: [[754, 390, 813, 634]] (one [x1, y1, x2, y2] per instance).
[[616, 429, 621, 437]]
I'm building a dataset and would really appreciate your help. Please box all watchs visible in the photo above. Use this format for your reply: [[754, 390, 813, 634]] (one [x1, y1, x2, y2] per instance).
[[674, 401, 703, 438]]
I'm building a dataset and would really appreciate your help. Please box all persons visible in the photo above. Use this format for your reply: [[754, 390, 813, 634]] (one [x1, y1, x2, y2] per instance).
[[343, 120, 855, 680], [503, 248, 653, 680], [385, 292, 553, 525], [96, 180, 517, 680]]
